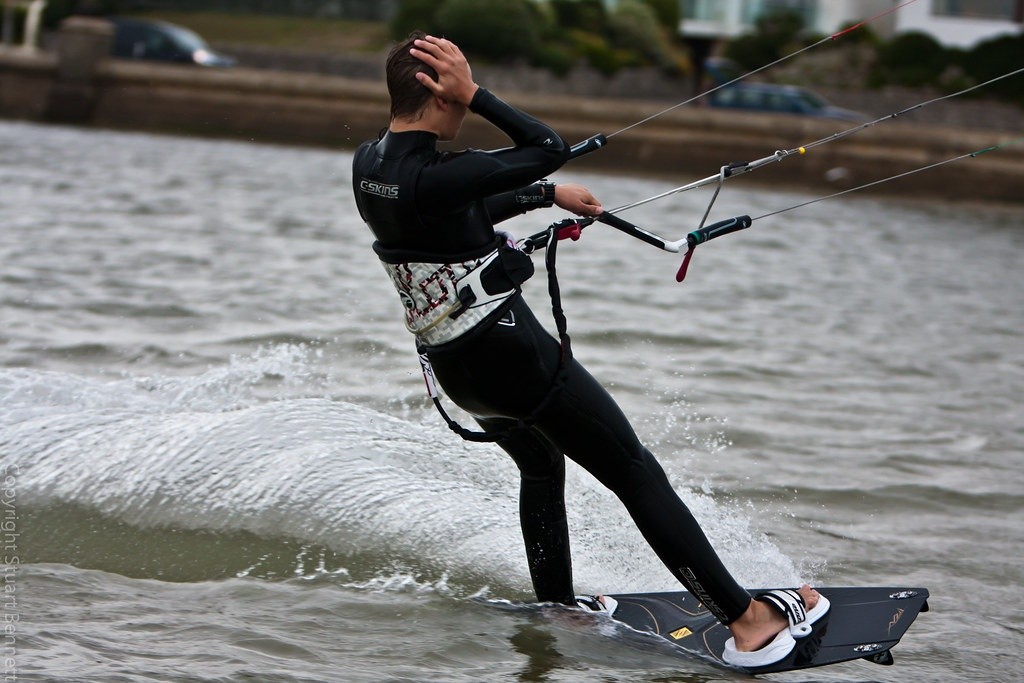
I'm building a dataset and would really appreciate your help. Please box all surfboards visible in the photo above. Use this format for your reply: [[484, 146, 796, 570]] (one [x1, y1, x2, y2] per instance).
[[512, 582, 935, 672]]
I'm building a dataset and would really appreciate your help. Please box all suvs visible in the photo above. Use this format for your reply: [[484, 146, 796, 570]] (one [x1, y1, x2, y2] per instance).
[[708, 80, 874, 121]]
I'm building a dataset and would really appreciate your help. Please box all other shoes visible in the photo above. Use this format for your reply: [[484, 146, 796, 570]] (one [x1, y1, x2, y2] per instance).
[[722, 589, 831, 666], [575, 594, 621, 619]]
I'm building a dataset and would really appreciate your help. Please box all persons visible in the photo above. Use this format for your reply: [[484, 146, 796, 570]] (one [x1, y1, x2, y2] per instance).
[[707, 42, 735, 87], [352, 31, 831, 667]]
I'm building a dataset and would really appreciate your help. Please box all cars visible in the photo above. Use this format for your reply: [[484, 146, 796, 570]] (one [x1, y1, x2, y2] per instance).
[[110, 18, 239, 68]]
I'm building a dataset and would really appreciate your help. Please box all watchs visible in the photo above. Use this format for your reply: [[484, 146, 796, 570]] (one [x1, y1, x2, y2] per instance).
[[540, 179, 556, 208]]
